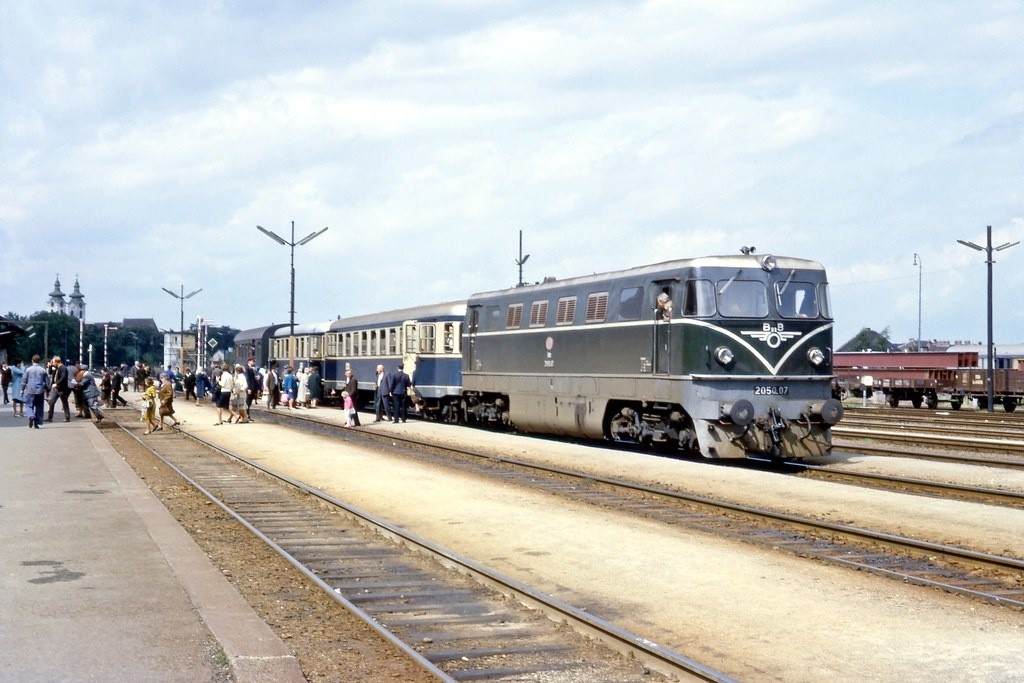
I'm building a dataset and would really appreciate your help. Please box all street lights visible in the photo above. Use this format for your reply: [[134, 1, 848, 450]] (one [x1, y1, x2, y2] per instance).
[[256, 221, 328, 369], [958, 226, 1020, 412], [161, 285, 203, 373]]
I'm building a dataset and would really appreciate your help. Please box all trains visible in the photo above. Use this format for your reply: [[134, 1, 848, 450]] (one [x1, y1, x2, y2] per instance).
[[235, 247, 847, 469], [832, 365, 1024, 413]]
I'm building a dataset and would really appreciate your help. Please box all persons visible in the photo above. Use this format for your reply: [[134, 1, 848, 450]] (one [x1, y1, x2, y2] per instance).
[[387, 363, 413, 424], [656, 292, 673, 322], [372, 363, 393, 422], [0, 354, 322, 435], [339, 390, 356, 428], [343, 368, 362, 427]]
